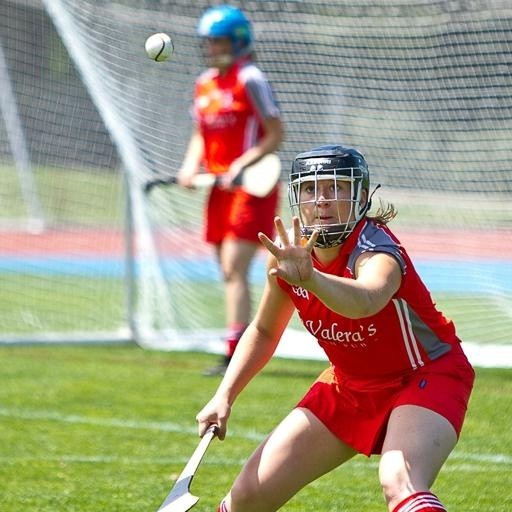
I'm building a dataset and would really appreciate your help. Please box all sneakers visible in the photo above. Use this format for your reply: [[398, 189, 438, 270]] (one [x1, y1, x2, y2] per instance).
[[203, 354, 229, 375]]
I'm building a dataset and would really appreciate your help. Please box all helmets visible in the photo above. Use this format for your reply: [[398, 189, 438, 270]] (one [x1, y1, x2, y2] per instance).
[[288, 145, 371, 248], [196, 5, 255, 67]]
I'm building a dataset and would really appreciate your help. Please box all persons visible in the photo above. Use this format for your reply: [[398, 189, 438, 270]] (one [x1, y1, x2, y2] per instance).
[[179, 5, 290, 375], [195, 147, 474, 511]]
